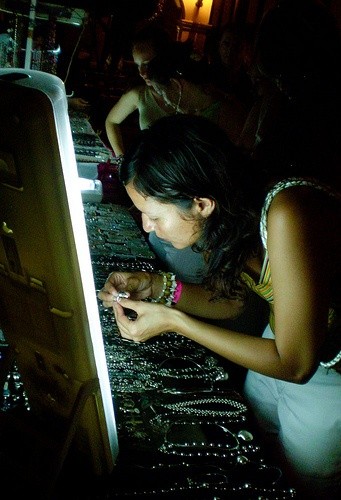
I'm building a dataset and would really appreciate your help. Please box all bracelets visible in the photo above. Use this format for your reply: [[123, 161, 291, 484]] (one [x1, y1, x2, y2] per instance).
[[115, 153, 127, 165], [145, 270, 182, 308]]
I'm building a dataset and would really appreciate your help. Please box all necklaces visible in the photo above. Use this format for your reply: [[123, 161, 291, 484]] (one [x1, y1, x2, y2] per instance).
[[68, 116, 304, 500], [81, 199, 298, 500], [152, 78, 182, 111]]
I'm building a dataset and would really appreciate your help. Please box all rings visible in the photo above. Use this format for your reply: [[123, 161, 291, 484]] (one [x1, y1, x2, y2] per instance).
[[115, 292, 128, 303]]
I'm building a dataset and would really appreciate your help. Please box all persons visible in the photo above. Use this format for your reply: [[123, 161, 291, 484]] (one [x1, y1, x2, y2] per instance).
[[96, 114, 341, 476], [208, 23, 260, 105], [0, 20, 100, 111], [104, 28, 176, 166], [145, 48, 260, 160]]
